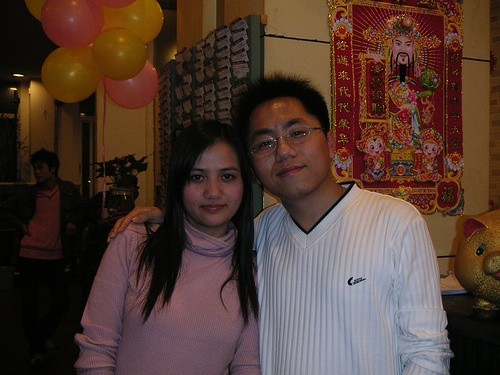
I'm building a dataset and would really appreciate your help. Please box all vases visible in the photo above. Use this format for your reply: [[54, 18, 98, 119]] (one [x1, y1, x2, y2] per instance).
[[108, 184, 135, 215]]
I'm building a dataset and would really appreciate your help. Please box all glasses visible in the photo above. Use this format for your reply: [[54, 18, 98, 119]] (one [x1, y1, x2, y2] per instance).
[[247, 126, 323, 157]]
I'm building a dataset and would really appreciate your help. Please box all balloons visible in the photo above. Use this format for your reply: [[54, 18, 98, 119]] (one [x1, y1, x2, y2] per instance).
[[39, 44, 100, 104], [40, 0, 103, 50], [92, 27, 146, 80], [25, 0, 45, 22], [102, 0, 136, 9], [103, 0, 164, 43], [104, 62, 158, 110]]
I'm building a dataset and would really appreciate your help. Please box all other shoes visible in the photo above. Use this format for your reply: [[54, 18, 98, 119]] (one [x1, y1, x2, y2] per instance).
[[31, 354, 44, 366], [45, 339, 59, 350]]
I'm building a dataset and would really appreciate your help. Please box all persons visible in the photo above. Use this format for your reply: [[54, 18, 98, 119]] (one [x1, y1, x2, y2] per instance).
[[108, 74, 452, 375], [0, 148, 87, 368], [72, 123, 261, 375]]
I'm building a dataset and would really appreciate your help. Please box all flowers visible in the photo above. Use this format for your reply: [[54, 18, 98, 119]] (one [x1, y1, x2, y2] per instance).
[[92, 151, 152, 188]]
